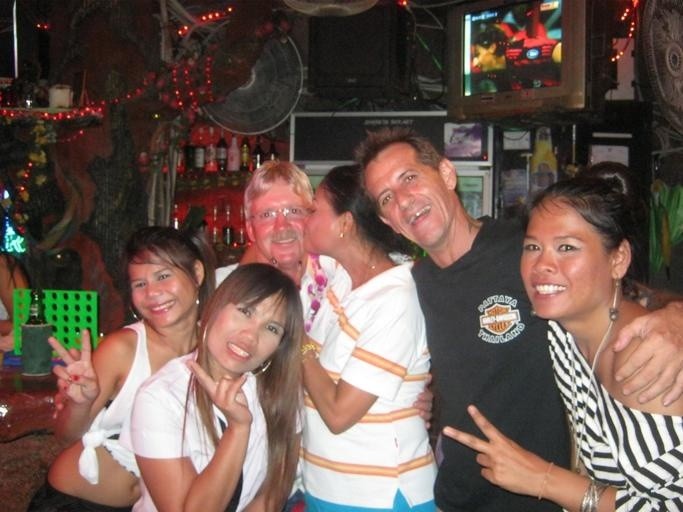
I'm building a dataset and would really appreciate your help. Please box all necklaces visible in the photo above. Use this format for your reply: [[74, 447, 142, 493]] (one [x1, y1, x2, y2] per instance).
[[562, 322, 612, 474], [283, 255, 329, 339], [361, 261, 378, 283]]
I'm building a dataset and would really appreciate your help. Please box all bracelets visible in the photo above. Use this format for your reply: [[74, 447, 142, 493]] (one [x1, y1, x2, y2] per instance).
[[536, 458, 553, 502], [300, 343, 315, 357], [576, 476, 610, 512]]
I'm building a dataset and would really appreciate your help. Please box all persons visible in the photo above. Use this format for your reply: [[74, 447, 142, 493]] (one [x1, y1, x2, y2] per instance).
[[118, 260, 310, 512], [350, 124, 683, 512], [287, 162, 442, 512], [23, 222, 216, 511], [193, 159, 434, 512], [0, 246, 32, 375], [443, 158, 683, 512]]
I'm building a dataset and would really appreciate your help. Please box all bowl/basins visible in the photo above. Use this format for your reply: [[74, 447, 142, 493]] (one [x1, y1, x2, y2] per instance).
[[20, 93, 40, 111]]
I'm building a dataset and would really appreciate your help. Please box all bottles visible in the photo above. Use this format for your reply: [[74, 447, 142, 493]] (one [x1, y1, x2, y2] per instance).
[[171, 126, 279, 248], [531, 127, 557, 198], [22, 287, 53, 383]]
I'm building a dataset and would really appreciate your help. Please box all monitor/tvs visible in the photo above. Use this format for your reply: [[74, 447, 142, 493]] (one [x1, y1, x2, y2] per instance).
[[445, 0, 594, 125]]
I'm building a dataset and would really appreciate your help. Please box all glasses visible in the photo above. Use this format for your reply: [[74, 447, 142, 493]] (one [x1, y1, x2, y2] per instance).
[[248, 206, 308, 221]]
